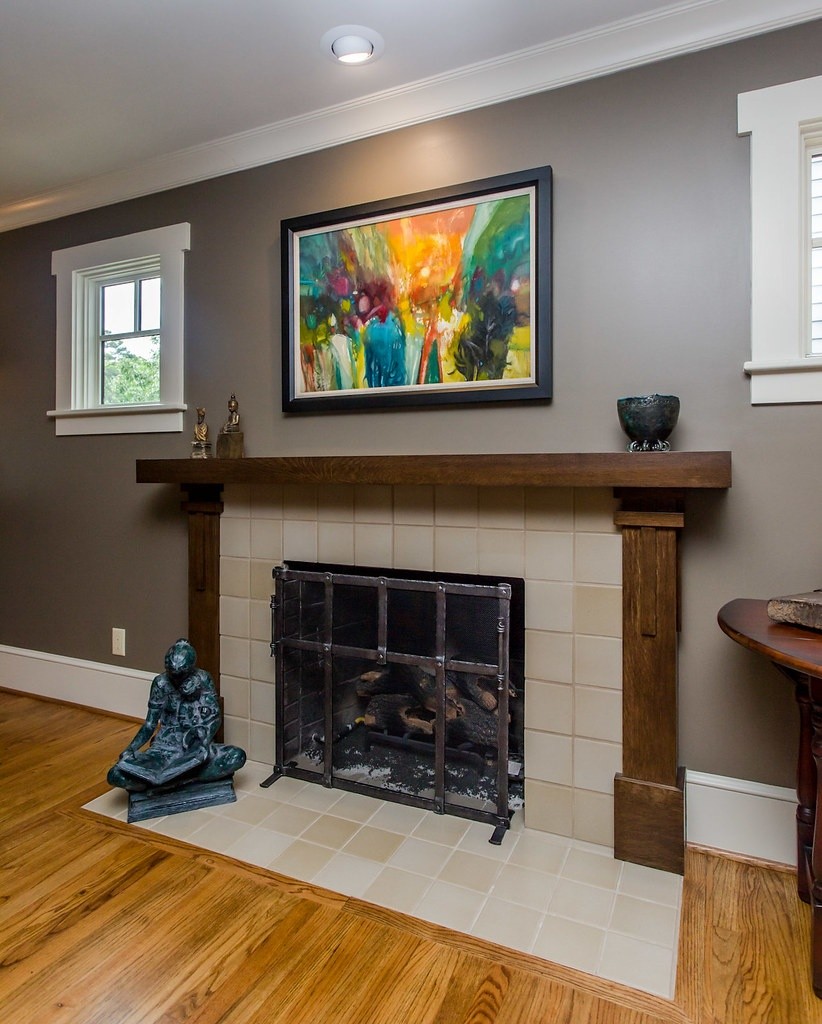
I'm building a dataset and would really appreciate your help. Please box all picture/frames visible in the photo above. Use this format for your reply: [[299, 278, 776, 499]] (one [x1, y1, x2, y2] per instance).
[[280, 165, 553, 414]]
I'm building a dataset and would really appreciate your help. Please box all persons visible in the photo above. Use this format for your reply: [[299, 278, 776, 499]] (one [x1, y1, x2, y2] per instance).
[[190, 406, 213, 441], [216, 394, 246, 432], [106, 640, 248, 796]]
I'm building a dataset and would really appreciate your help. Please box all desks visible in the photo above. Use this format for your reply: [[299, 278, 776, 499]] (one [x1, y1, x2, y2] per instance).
[[717, 598, 822, 1000]]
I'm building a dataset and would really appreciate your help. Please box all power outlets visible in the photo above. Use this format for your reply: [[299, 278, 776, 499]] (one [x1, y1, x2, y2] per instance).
[[112, 628, 126, 657]]
